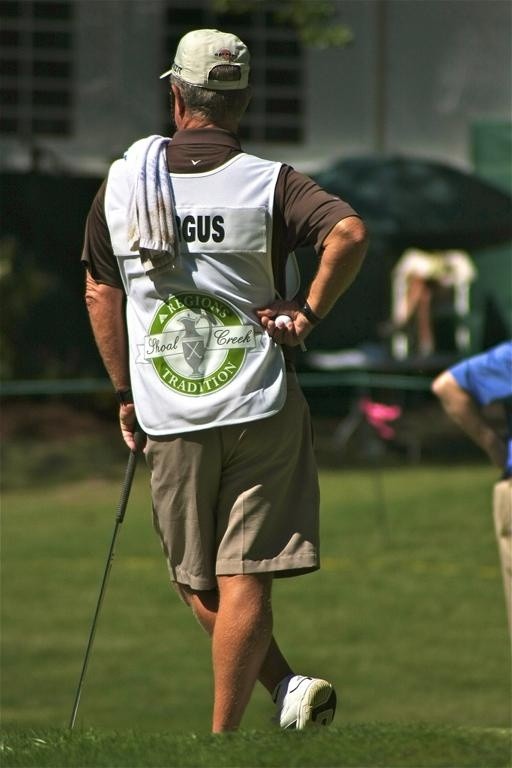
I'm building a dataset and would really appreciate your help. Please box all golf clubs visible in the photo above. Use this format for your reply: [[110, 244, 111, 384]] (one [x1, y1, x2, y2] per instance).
[[30, 426, 145, 748]]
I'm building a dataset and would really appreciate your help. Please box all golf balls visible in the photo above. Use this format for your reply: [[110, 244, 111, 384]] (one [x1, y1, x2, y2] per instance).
[[275, 316, 291, 328]]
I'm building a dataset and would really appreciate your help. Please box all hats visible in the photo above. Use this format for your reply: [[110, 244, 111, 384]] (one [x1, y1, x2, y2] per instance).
[[157, 28, 253, 89]]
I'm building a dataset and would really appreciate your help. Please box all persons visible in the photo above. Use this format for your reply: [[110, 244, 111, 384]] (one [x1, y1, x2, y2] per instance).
[[431, 336, 510, 635], [78, 26, 368, 731]]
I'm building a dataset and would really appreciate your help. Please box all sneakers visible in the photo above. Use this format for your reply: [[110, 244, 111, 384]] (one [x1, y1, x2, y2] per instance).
[[272, 672, 337, 735]]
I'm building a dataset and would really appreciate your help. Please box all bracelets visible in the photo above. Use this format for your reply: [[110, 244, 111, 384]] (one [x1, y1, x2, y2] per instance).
[[113, 389, 132, 405], [299, 296, 323, 326]]
